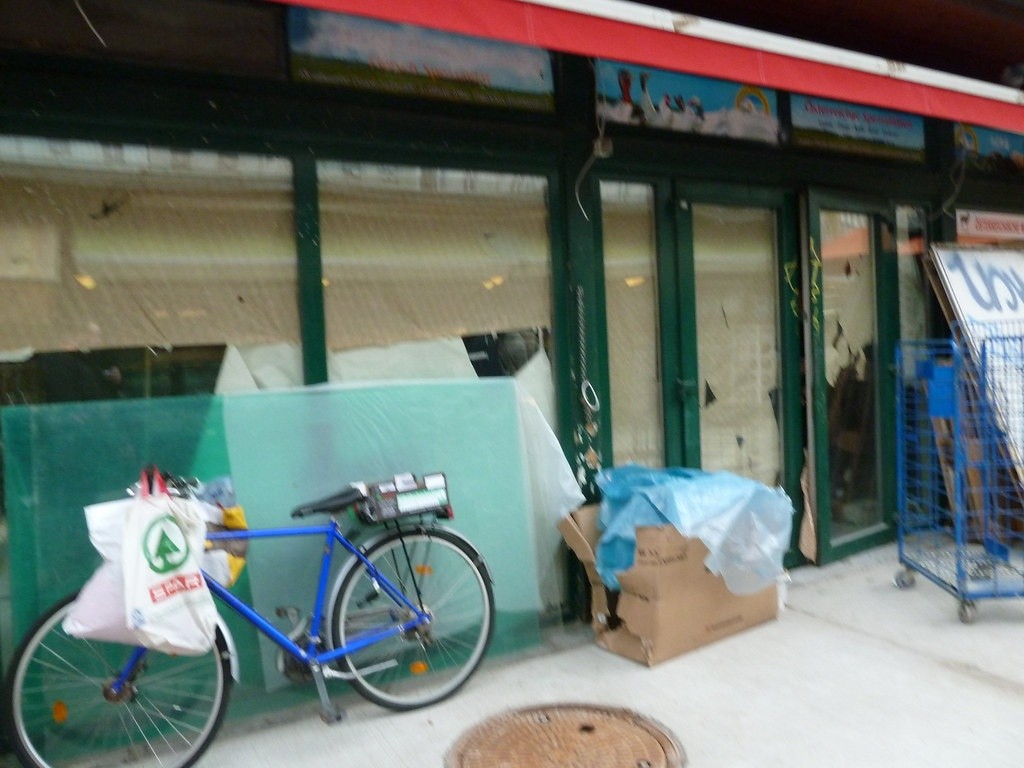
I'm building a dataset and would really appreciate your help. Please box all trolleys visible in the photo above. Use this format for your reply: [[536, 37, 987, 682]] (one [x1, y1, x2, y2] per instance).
[[893, 320, 1024, 624]]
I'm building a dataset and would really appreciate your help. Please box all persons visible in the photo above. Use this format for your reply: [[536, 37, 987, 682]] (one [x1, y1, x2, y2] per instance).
[[597, 67, 706, 134]]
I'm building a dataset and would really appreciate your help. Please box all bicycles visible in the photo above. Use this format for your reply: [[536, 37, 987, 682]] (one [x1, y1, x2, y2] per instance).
[[4, 463, 495, 768]]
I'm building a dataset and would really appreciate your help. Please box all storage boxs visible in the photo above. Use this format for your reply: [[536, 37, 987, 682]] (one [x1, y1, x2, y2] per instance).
[[551, 468, 778, 668], [343, 470, 451, 527]]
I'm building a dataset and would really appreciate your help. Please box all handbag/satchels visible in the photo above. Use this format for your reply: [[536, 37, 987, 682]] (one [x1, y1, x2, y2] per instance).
[[180, 477, 248, 589], [122, 465, 217, 658], [61, 489, 208, 655]]
